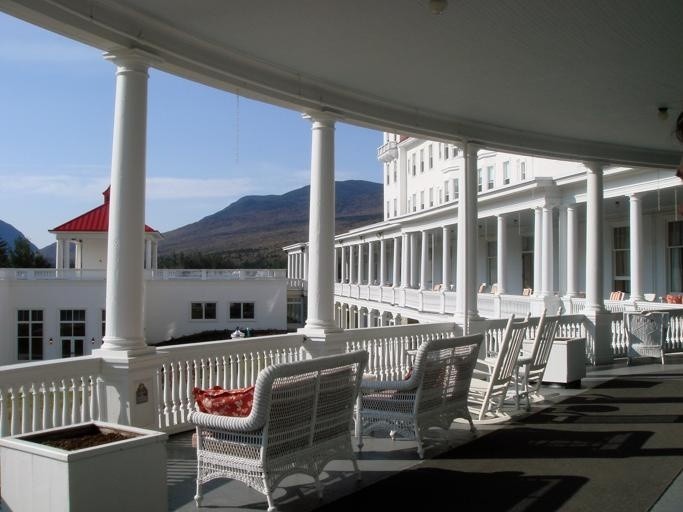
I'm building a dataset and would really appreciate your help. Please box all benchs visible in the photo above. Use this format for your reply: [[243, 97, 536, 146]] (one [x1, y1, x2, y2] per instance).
[[187, 347, 371, 512], [349, 330, 485, 464]]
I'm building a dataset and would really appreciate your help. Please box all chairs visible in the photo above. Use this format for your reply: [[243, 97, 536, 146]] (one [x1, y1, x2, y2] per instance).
[[402, 310, 533, 422], [622, 311, 669, 366], [449, 305, 565, 399], [428, 281, 655, 300]]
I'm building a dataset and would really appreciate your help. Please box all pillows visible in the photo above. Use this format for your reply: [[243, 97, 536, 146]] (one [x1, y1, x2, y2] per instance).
[[389, 360, 446, 403], [190, 385, 258, 420]]
